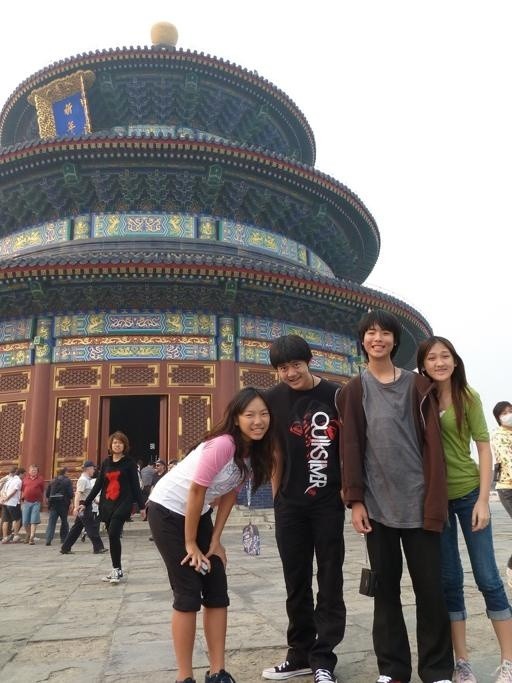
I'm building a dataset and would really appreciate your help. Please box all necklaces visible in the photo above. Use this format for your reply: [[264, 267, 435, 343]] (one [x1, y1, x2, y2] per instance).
[[288, 373, 315, 422]]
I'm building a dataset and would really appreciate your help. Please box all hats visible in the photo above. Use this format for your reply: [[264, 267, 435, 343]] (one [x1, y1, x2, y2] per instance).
[[84, 460, 96, 467], [155, 459, 165, 464]]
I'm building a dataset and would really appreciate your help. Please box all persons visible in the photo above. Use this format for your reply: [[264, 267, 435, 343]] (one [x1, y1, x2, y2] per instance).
[[489, 400, 512, 589], [416, 336, 512, 682], [145, 386, 277, 683], [0, 430, 180, 584], [261, 333, 351, 683], [338, 309, 454, 683]]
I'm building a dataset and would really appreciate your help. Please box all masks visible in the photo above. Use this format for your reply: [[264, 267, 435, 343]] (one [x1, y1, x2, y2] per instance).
[[498, 413, 512, 426]]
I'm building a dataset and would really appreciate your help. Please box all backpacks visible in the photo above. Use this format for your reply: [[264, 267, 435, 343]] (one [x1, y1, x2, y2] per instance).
[[47, 476, 67, 502]]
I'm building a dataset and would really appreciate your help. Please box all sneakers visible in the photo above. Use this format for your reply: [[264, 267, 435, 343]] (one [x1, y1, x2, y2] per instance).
[[1, 531, 123, 585]]
